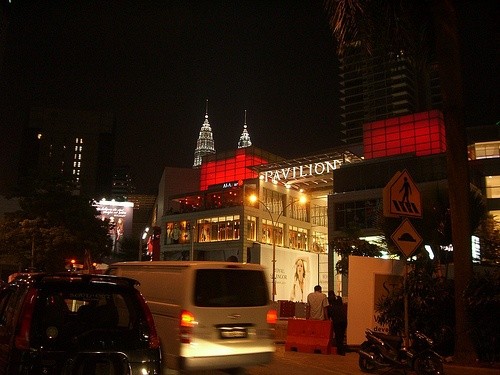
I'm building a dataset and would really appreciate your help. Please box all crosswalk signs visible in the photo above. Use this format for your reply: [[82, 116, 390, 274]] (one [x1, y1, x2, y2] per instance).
[[388, 168, 424, 220]]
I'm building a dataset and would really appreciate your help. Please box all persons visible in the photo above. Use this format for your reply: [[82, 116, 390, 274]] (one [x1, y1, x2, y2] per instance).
[[291, 259, 309, 304], [306, 285, 330, 321], [328, 292, 348, 356]]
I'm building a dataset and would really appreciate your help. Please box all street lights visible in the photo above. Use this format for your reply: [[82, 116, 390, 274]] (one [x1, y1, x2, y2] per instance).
[[247, 188, 306, 301]]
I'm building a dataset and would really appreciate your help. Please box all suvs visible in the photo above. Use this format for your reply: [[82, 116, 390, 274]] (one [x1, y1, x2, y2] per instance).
[[0, 272, 163, 375]]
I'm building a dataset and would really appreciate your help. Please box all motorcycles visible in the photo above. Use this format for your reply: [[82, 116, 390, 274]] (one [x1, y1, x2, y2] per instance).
[[357, 326, 446, 375]]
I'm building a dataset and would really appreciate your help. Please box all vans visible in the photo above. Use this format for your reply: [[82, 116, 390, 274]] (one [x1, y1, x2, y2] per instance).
[[97, 260, 278, 371]]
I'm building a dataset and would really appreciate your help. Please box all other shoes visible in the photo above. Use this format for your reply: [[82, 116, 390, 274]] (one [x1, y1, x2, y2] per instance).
[[336, 351, 345, 355]]
